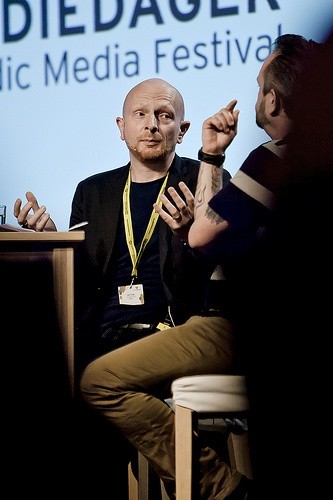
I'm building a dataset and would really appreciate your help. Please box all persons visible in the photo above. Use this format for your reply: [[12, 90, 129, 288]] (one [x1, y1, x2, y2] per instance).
[[81, 34, 323, 500], [14, 78, 231, 360]]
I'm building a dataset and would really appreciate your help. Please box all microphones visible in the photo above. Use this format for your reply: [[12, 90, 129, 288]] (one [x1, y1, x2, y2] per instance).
[[127, 144, 136, 151]]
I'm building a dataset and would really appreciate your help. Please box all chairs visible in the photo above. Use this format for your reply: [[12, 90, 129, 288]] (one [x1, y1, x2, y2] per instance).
[[138, 371, 249, 500]]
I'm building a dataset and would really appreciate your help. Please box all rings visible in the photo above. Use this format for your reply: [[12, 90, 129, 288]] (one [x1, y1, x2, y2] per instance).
[[20, 223, 28, 227], [174, 215, 181, 219]]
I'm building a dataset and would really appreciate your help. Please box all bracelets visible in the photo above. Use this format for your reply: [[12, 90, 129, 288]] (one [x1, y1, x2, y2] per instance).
[[198, 147, 225, 167]]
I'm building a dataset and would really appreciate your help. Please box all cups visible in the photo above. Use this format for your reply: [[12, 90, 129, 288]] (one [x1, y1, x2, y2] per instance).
[[0, 204, 6, 226]]
